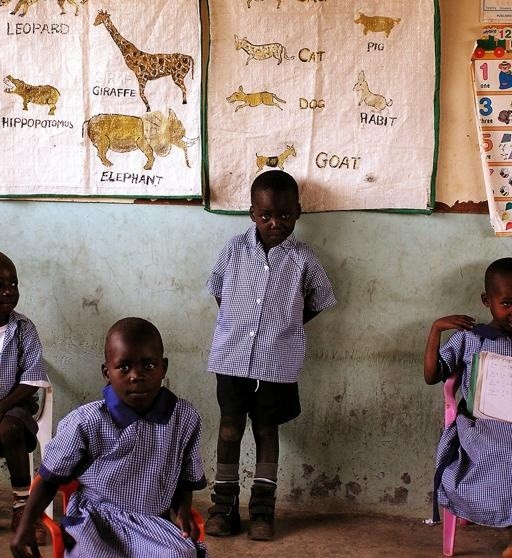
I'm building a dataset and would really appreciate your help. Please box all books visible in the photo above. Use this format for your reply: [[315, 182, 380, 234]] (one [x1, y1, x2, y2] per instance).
[[467, 349, 511, 424]]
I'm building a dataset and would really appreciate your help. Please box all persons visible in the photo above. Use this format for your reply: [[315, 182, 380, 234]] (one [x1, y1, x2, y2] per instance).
[[1, 251, 47, 547], [195, 168, 339, 542], [422, 257, 511, 536], [9, 315, 211, 558]]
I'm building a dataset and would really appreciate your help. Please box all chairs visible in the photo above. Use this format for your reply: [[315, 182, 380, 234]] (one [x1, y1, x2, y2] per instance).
[[27, 374, 54, 527], [441, 378, 467, 557], [28, 467, 205, 558]]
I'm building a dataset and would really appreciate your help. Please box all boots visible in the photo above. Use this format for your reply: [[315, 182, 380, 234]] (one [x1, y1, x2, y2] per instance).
[[248, 478, 277, 540], [11, 493, 48, 546], [205, 477, 240, 536]]
